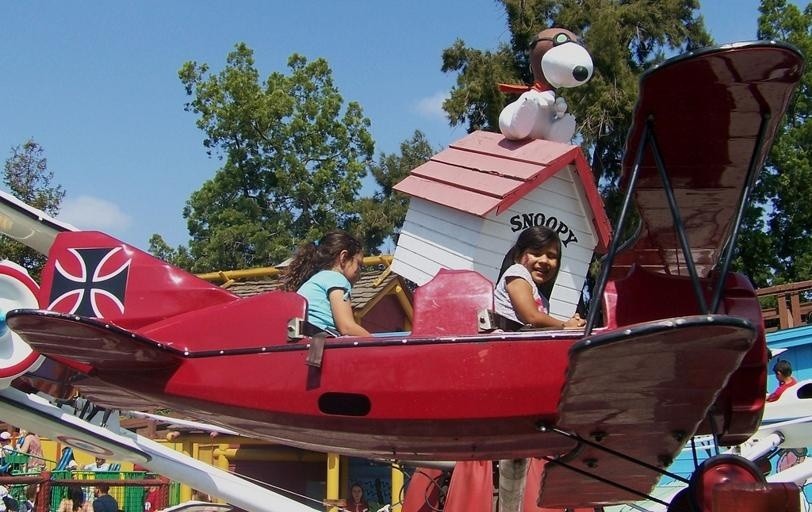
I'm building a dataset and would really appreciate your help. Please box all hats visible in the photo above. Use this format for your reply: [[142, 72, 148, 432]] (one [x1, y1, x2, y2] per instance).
[[1, 431, 13, 439]]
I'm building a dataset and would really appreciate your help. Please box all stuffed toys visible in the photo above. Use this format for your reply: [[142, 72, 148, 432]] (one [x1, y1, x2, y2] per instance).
[[498, 28, 593, 144]]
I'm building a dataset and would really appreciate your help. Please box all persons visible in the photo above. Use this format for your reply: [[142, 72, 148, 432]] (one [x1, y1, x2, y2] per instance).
[[765, 359, 796, 401], [342, 483, 369, 512], [491, 226, 587, 331], [284, 230, 371, 344], [0, 422, 162, 512]]
